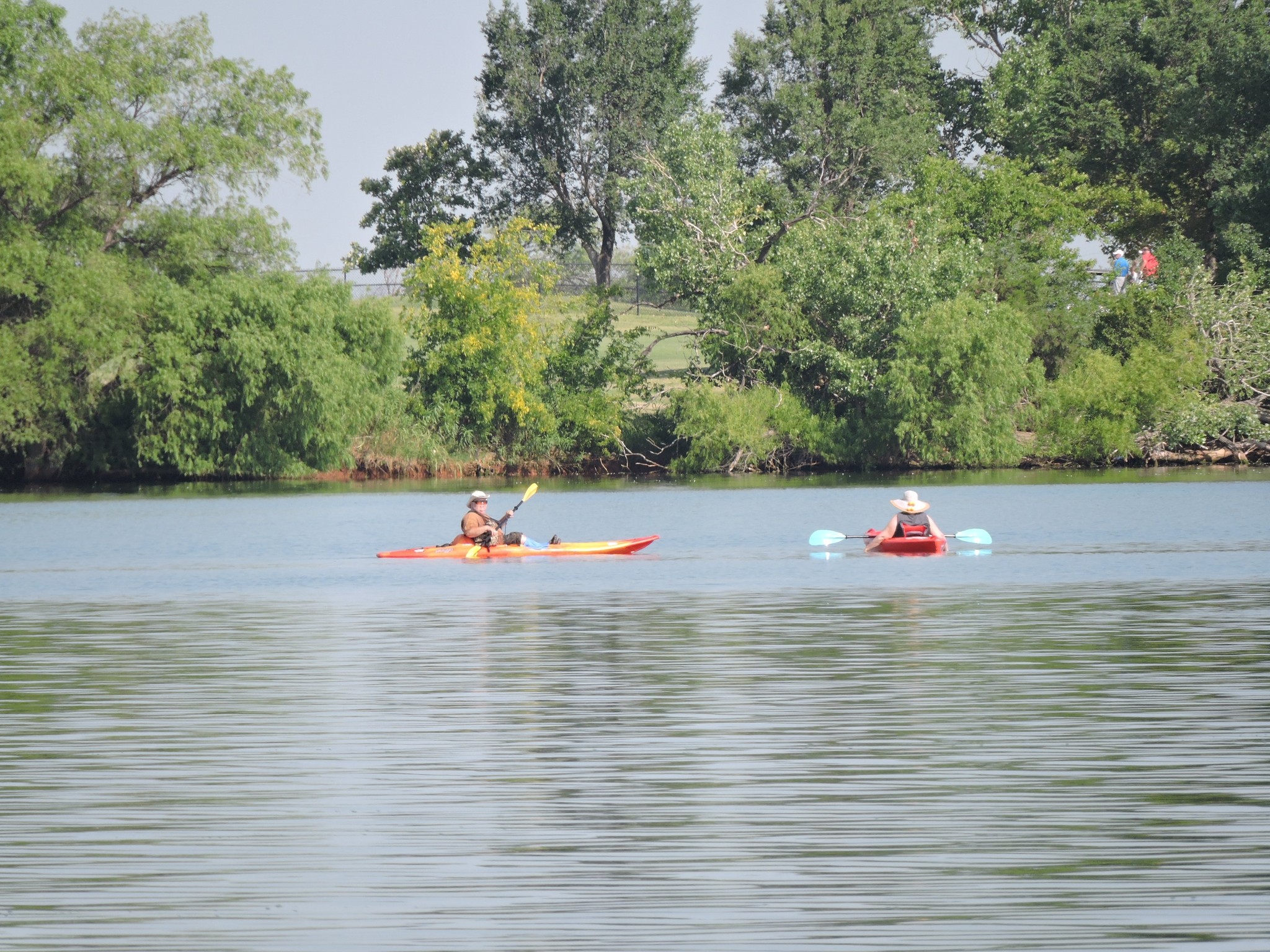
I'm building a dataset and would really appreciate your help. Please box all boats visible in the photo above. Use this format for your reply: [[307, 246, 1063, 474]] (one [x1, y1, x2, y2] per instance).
[[375, 534, 661, 558], [863, 528, 947, 557]]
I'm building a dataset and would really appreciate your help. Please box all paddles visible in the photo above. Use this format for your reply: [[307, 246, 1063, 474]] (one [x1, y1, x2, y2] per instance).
[[809, 526, 992, 546], [809, 548, 993, 560], [466, 483, 539, 559]]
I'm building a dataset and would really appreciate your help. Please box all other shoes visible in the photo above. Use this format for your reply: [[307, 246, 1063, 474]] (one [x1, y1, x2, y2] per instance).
[[549, 535, 557, 544], [556, 538, 561, 544]]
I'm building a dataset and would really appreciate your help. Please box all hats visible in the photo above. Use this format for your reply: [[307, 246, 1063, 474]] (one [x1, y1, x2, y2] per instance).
[[467, 491, 490, 508], [890, 490, 929, 513], [1113, 250, 1124, 256]]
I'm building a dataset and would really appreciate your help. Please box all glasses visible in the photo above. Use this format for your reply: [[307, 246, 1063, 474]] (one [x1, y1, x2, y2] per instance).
[[472, 500, 488, 505]]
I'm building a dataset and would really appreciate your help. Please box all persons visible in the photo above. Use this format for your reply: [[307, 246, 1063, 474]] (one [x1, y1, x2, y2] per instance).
[[461, 490, 561, 551], [864, 490, 948, 553]]
